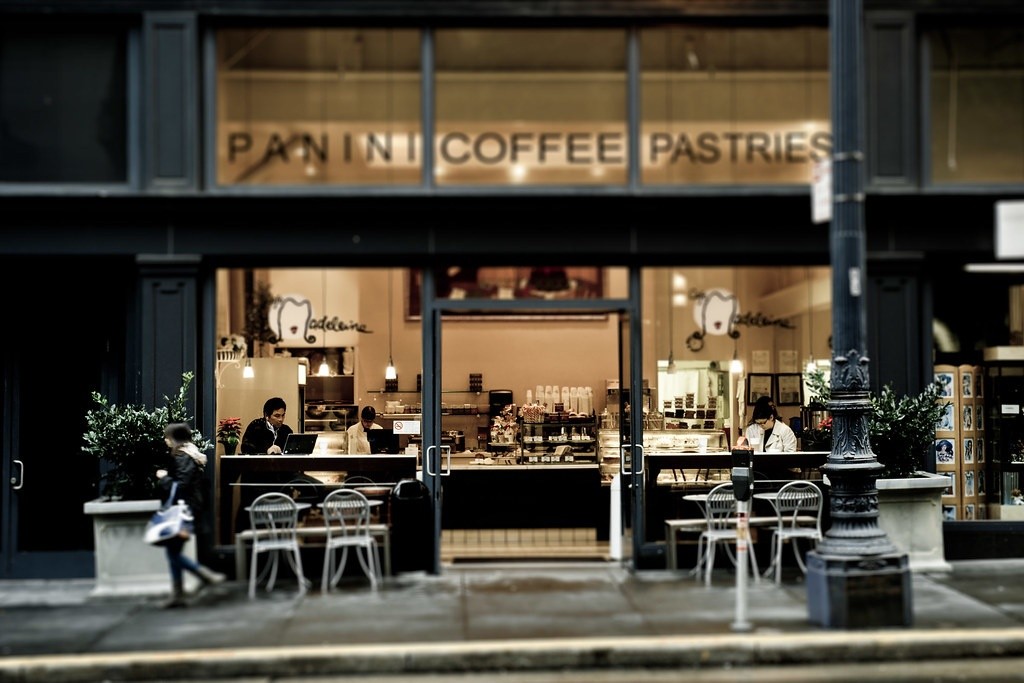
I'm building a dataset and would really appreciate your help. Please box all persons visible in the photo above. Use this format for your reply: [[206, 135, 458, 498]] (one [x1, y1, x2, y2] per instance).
[[966, 506, 973, 520], [963, 406, 972, 429], [977, 471, 986, 494], [745, 396, 797, 453], [934, 373, 952, 396], [976, 440, 982, 460], [156, 419, 225, 610], [977, 406, 983, 429], [939, 407, 949, 429], [964, 472, 973, 496], [936, 440, 953, 462], [965, 440, 972, 460], [963, 375, 971, 395], [239, 397, 293, 582], [346, 406, 384, 455]]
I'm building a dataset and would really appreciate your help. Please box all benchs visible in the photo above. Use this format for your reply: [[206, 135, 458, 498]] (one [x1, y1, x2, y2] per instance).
[[235, 525, 389, 586], [664, 516, 817, 569]]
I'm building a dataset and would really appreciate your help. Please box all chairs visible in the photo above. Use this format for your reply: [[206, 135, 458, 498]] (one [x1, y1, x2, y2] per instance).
[[320, 489, 383, 590], [249, 493, 306, 598], [770, 480, 824, 586], [699, 482, 761, 590]]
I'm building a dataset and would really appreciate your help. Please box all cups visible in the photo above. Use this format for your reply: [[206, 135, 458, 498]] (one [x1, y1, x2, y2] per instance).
[[562, 386, 570, 411], [552, 386, 559, 412], [545, 386, 552, 413], [536, 385, 544, 407], [576, 386, 593, 416], [570, 387, 577, 414], [526, 390, 532, 404]]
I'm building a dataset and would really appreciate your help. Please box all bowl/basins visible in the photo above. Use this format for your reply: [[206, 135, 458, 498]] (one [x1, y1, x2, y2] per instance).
[[551, 412, 558, 415], [663, 393, 717, 408]]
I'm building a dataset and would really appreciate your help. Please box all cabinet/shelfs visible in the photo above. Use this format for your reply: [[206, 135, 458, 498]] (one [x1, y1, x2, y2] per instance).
[[664, 407, 723, 432], [520, 416, 599, 465]]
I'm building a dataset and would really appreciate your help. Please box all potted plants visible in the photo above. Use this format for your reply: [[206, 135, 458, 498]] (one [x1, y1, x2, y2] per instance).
[[81, 373, 208, 598], [806, 372, 953, 574]]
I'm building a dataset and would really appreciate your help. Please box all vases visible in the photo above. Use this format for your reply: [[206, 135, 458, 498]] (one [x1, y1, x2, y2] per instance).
[[218, 438, 238, 455]]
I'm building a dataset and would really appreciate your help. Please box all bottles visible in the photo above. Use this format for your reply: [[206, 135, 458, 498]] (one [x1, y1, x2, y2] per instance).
[[601, 408, 612, 430], [764, 388, 769, 396], [781, 387, 787, 402]]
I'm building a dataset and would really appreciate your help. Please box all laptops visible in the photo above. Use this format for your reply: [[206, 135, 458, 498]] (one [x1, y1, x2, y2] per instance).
[[270, 433, 319, 455], [368, 428, 400, 454]]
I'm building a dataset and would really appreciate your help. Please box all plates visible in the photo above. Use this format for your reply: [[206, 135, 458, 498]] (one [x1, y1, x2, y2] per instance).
[[655, 444, 672, 448], [683, 444, 699, 448]]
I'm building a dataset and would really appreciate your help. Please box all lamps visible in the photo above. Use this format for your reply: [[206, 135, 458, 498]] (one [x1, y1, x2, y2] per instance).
[[317, 272, 331, 379], [385, 270, 398, 380], [243, 357, 254, 378]]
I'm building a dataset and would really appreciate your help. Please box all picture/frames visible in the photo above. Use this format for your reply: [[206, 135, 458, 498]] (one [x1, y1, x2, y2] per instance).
[[964, 502, 975, 521], [975, 435, 987, 466], [943, 503, 960, 521], [935, 436, 960, 467], [976, 403, 984, 433], [962, 436, 975, 466], [937, 467, 960, 502], [961, 404, 975, 434], [962, 467, 975, 497], [975, 372, 985, 401], [976, 470, 986, 498], [960, 366, 975, 400], [934, 365, 958, 398], [937, 402, 960, 437]]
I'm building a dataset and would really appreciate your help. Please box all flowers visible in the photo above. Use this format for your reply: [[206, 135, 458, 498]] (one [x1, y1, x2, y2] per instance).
[[219, 416, 242, 435]]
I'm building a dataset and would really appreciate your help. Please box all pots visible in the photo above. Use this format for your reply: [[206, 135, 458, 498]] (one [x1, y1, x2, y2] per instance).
[[329, 421, 353, 431], [305, 409, 329, 420], [331, 407, 357, 418], [305, 426, 326, 431]]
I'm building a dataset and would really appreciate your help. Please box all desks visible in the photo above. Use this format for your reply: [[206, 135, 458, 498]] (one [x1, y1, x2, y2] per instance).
[[752, 493, 821, 578], [245, 504, 313, 587], [682, 495, 743, 577], [367, 389, 491, 416], [318, 500, 384, 586]]
